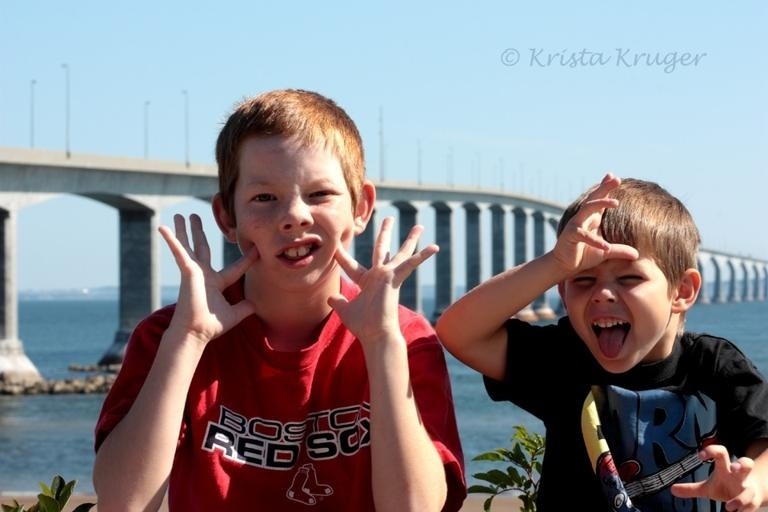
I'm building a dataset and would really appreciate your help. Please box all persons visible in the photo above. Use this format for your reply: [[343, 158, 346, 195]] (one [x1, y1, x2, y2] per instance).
[[92, 88, 466, 511], [435, 170, 768, 511]]
